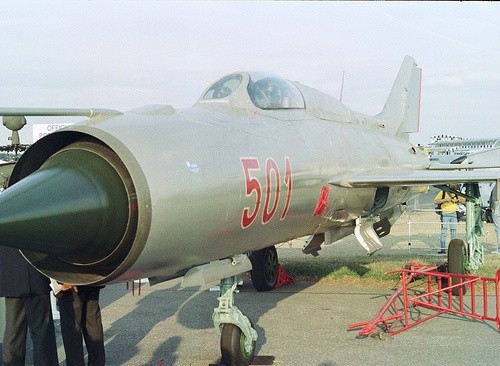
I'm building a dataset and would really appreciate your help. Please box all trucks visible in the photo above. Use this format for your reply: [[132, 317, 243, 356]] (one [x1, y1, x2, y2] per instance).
[[435, 182, 496, 221]]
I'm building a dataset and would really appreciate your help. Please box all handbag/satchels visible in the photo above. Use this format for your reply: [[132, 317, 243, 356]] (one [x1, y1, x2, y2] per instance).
[[435, 203, 442, 216]]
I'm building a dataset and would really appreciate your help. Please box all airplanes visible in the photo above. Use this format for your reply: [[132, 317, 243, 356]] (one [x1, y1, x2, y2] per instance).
[[0, 55, 500, 366]]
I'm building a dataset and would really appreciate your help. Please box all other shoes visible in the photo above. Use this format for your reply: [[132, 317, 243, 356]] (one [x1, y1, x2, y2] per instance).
[[437, 250, 446, 254]]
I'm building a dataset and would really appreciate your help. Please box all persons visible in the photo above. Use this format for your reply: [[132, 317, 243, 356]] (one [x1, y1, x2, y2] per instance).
[[0, 245, 60, 366], [433, 183, 465, 254], [49, 279, 106, 366]]
